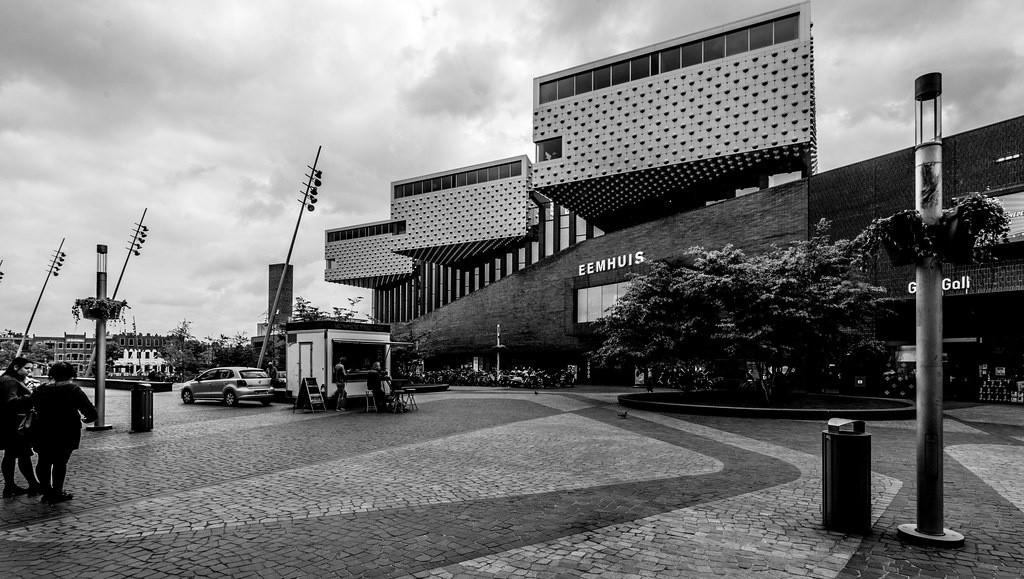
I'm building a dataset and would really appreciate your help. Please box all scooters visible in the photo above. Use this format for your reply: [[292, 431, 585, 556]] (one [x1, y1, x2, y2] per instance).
[[406, 367, 576, 390]]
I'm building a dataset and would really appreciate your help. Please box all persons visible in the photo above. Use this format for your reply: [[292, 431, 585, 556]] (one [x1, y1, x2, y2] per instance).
[[503, 366, 533, 375], [30, 361, 98, 502], [334, 357, 351, 411], [544, 150, 561, 161], [367, 361, 394, 413], [491, 366, 497, 376], [568, 366, 574, 387], [746, 368, 755, 383], [149, 370, 181, 383], [0, 357, 45, 498], [266, 362, 278, 388]]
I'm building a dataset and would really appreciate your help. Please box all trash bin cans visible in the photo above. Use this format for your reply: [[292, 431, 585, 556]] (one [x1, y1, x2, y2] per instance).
[[822, 418, 871, 532], [131, 384, 154, 431]]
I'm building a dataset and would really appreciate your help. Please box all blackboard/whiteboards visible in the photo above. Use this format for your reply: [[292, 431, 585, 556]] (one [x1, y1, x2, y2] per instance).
[[297, 377, 327, 414]]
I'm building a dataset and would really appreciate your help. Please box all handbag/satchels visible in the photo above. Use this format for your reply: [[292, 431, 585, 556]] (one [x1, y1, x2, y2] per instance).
[[18, 409, 38, 438]]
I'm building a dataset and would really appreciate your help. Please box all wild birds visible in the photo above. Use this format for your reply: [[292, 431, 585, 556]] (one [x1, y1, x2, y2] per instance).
[[618, 411, 627, 419], [535, 390, 540, 395]]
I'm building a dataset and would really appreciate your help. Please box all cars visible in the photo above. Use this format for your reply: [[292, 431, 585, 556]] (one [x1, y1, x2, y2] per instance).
[[69, 362, 85, 377], [181, 367, 275, 407]]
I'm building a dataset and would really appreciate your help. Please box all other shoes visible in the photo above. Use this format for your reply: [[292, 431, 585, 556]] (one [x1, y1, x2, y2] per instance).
[[27, 483, 42, 497], [41, 491, 74, 504], [335, 409, 340, 412], [3, 486, 28, 497], [340, 408, 345, 411]]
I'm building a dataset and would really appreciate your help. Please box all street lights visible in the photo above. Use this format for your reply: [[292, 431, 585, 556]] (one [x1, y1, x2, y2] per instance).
[[84, 207, 150, 375], [894, 71, 967, 549], [14, 237, 66, 358], [85, 244, 114, 431], [256, 145, 323, 368]]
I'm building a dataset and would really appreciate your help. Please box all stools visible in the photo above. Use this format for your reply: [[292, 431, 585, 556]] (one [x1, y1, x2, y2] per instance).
[[395, 389, 418, 413], [365, 390, 377, 412]]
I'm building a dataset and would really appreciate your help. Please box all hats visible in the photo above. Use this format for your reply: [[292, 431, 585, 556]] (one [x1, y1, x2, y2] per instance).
[[268, 362, 273, 365]]
